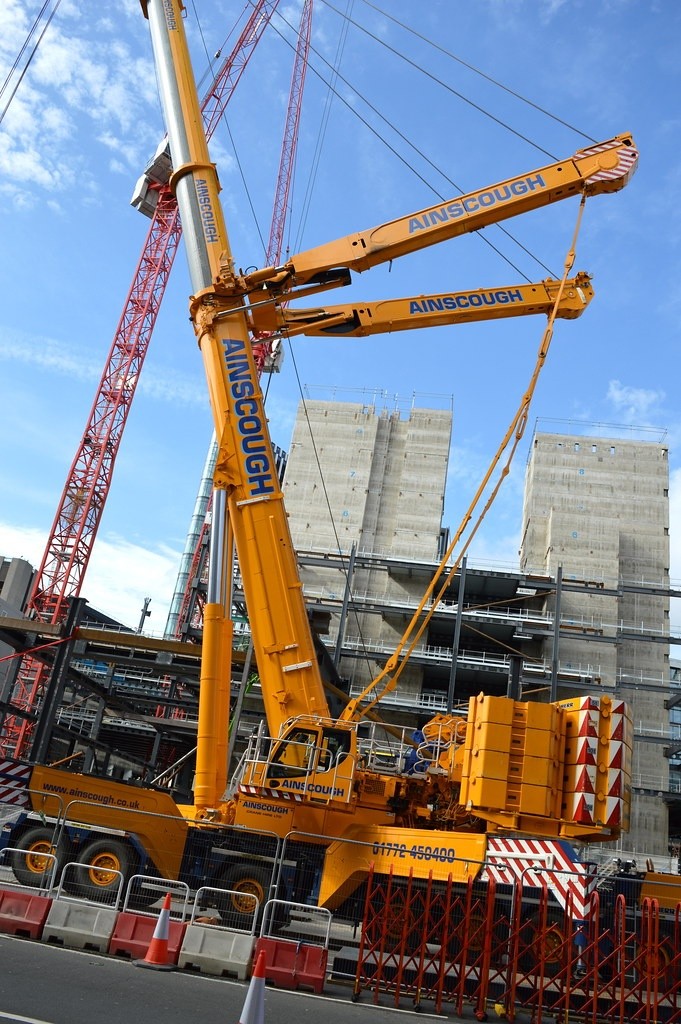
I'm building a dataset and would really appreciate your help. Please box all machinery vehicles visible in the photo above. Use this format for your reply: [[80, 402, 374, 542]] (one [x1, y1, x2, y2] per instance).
[[10, 1, 640, 977]]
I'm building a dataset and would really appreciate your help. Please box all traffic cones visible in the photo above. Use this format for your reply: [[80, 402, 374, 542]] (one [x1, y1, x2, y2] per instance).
[[235, 950, 268, 1024], [132, 891, 177, 973]]
